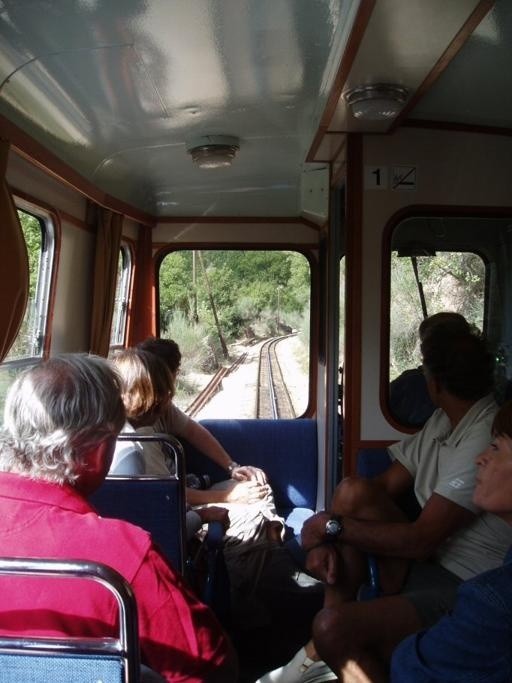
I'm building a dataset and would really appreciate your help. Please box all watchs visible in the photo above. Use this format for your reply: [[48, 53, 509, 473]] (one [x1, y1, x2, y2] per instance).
[[323, 514, 346, 542]]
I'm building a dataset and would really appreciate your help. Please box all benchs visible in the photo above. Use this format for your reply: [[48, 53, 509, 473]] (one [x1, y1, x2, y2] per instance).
[[177, 417, 316, 541]]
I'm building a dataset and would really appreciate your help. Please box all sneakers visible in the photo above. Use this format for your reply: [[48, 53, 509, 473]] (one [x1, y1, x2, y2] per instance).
[[253, 647, 338, 683]]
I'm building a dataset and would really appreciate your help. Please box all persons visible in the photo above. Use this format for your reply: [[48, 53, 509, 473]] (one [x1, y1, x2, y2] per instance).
[[253, 325, 508, 682], [103, 346, 229, 545], [311, 405, 511, 682], [137, 339, 285, 570], [0, 352, 241, 682], [388, 312, 470, 430]]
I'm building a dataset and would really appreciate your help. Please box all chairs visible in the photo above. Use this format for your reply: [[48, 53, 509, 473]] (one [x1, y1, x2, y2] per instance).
[[352, 447, 411, 601], [88, 435, 187, 582], [0, 556, 140, 683]]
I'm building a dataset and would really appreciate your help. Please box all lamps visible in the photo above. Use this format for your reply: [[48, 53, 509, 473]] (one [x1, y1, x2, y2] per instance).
[[185, 136, 240, 169], [344, 83, 409, 120]]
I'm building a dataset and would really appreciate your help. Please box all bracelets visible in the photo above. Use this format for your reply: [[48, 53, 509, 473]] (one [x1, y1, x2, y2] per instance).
[[225, 462, 239, 473]]
